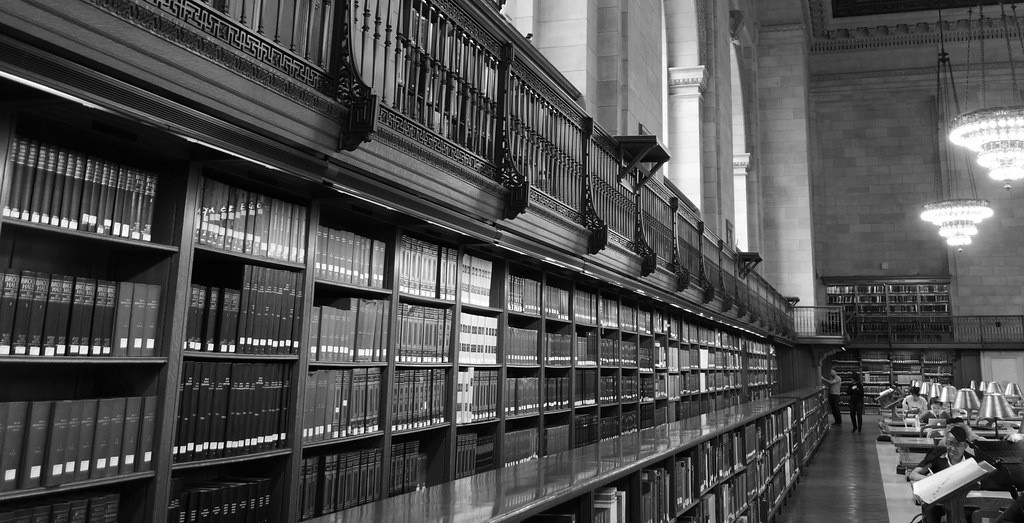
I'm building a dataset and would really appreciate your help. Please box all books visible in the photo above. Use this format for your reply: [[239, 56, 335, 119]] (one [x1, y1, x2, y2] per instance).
[[0, 134, 952, 523]]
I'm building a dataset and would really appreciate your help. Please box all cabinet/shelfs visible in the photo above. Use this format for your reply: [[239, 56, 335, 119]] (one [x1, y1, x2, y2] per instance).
[[0, 136, 829, 523], [826, 283, 952, 338]]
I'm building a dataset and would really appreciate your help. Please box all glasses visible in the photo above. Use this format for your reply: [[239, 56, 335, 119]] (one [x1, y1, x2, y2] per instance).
[[946, 440, 964, 448]]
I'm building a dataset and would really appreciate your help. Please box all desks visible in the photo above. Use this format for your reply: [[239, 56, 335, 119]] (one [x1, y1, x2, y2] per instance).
[[879, 406, 1024, 523]]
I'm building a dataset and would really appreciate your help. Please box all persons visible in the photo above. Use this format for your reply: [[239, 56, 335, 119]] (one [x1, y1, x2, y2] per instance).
[[847, 374, 864, 432], [914, 396, 951, 428], [902, 386, 928, 412], [821, 369, 842, 427], [908, 423, 983, 523]]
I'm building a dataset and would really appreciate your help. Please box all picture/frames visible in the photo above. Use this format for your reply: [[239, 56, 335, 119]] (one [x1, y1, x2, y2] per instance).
[[830, 349, 955, 415]]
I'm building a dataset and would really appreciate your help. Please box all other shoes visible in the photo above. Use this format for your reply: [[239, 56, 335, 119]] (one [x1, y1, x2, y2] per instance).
[[858, 425, 861, 432], [831, 422, 841, 425], [851, 425, 857, 433]]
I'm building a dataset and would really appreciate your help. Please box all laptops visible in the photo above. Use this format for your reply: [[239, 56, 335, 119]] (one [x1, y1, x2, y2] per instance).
[[929, 419, 947, 426]]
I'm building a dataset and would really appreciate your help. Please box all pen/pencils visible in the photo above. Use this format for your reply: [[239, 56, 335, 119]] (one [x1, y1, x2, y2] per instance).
[[926, 467, 935, 475]]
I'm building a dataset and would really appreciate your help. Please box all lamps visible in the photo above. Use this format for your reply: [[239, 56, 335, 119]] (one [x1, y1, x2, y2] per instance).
[[919, 52, 991, 248], [965, 379, 1022, 407], [909, 378, 1014, 438], [947, 0, 1024, 192]]
